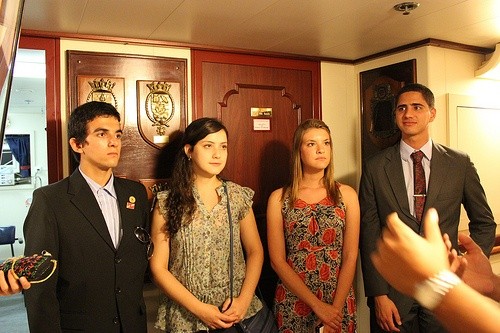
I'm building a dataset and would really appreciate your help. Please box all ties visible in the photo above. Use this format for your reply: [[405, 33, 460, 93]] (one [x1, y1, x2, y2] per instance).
[[410, 151, 426, 224]]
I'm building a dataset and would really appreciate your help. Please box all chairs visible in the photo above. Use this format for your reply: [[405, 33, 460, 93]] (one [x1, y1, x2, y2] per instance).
[[0, 225, 23, 257]]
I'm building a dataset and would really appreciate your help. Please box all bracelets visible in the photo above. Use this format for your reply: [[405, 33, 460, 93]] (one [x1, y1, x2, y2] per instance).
[[414, 271, 464, 310]]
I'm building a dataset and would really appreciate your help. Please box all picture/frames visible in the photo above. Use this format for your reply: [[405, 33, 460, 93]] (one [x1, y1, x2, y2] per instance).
[[359, 58, 417, 174]]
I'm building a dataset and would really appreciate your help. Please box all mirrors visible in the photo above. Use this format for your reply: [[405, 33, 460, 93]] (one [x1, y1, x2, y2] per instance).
[[0, 129, 37, 191]]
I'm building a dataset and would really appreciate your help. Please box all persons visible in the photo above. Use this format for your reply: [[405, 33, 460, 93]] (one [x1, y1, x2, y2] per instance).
[[358, 83, 497, 333], [266, 119, 361, 333], [371, 209, 500, 333], [0, 269, 31, 297], [149, 117, 265, 333], [22, 101, 150, 333]]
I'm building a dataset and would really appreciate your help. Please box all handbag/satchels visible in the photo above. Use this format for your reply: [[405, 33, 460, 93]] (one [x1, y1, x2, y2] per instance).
[[215, 297, 276, 333]]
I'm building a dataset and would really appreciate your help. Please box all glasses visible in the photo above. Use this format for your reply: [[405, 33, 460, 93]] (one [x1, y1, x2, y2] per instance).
[[134, 226, 154, 259]]
